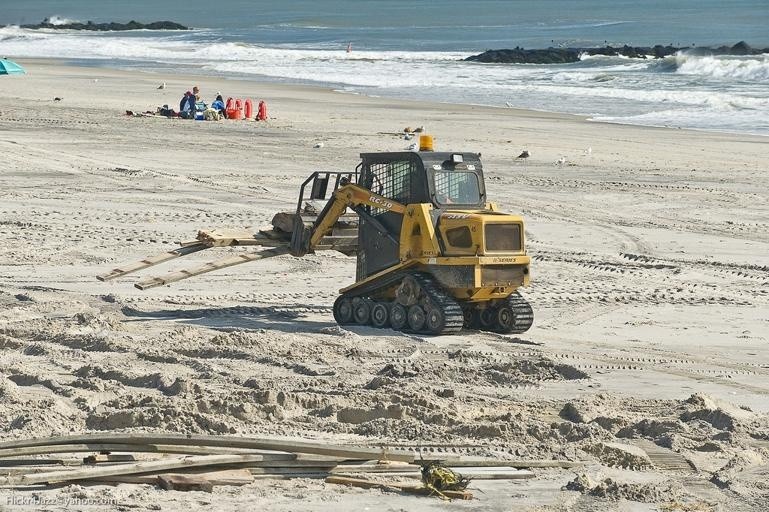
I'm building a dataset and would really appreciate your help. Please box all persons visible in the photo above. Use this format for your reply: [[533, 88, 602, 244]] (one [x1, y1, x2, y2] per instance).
[[178, 87, 229, 120]]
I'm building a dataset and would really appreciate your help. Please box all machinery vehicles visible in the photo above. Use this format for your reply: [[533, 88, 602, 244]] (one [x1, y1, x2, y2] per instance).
[[290, 136, 533, 334]]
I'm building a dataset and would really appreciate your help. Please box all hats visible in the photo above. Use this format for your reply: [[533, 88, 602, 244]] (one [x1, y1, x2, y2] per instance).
[[186, 90, 192, 96]]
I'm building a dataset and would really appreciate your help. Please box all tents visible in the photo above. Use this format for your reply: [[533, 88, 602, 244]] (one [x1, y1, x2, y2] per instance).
[[0, 58, 27, 76]]
